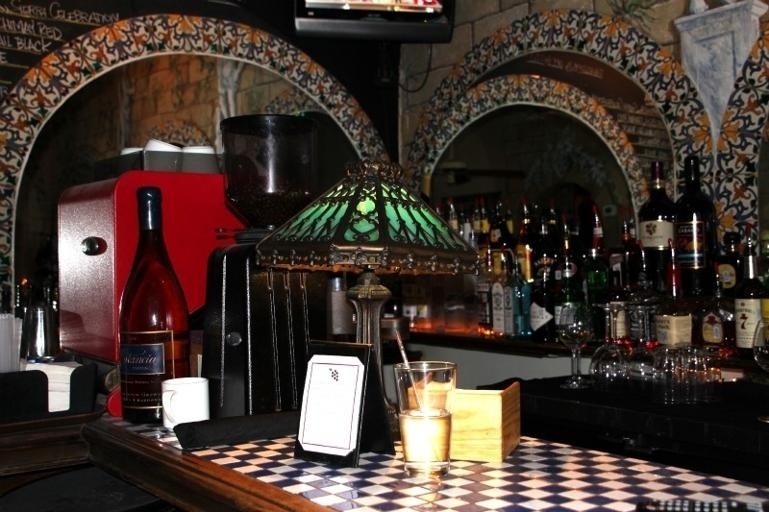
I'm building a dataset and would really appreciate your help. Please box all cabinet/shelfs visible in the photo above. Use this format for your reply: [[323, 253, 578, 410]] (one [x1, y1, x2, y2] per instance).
[[0, 407, 769, 511], [399, 290, 768, 483]]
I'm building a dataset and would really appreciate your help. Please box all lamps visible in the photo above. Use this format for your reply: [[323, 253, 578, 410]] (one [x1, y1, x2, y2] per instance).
[[253, 146, 481, 464]]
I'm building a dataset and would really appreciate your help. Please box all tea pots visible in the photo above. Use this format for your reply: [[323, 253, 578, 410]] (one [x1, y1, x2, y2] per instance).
[[20, 301, 58, 363]]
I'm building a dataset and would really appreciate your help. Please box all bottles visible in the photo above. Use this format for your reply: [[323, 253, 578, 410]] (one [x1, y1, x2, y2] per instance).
[[672, 154, 716, 283], [627, 250, 661, 345], [116, 185, 191, 425], [713, 237, 742, 300], [698, 279, 734, 350], [446, 197, 610, 337], [761, 231, 769, 348], [654, 244, 695, 347], [638, 160, 673, 274], [605, 269, 628, 344], [618, 217, 637, 287], [731, 223, 763, 353]]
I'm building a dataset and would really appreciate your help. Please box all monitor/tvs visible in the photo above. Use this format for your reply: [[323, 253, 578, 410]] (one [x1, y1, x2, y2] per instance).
[[287, 0, 454, 46]]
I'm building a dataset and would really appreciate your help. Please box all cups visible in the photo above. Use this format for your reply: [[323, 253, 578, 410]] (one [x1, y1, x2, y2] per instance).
[[627, 299, 662, 382], [161, 377, 210, 432], [394, 360, 457, 478], [663, 382, 713, 406], [753, 319, 769, 423], [182, 146, 220, 175], [589, 303, 629, 385], [559, 302, 596, 388], [656, 344, 712, 379], [142, 138, 181, 170]]
